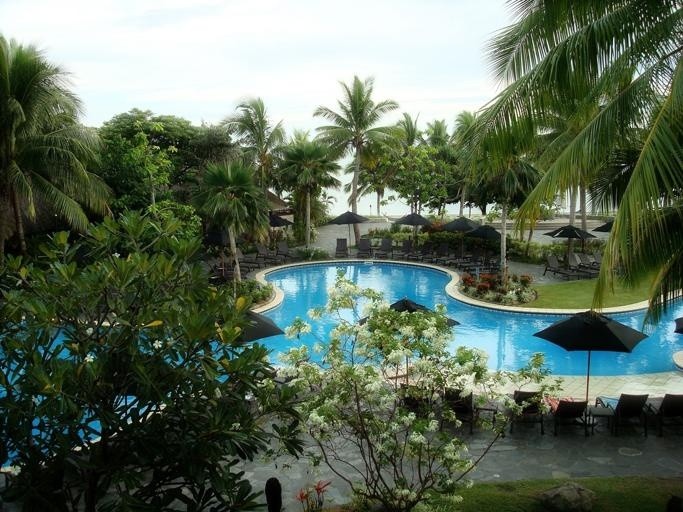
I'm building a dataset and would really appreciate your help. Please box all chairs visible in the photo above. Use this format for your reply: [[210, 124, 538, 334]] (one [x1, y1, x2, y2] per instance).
[[334, 237, 509, 279], [200, 240, 307, 283], [541, 251, 603, 281], [397, 381, 682, 438]]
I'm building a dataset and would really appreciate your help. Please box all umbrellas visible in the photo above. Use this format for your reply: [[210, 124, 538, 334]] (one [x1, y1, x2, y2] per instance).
[[270, 215, 294, 237], [592, 220, 614, 232], [210, 310, 285, 359], [395, 213, 432, 240], [357, 296, 460, 384], [327, 211, 369, 253], [674, 317, 683, 334], [542, 225, 598, 255], [533, 307, 649, 401], [440, 217, 501, 258]]
